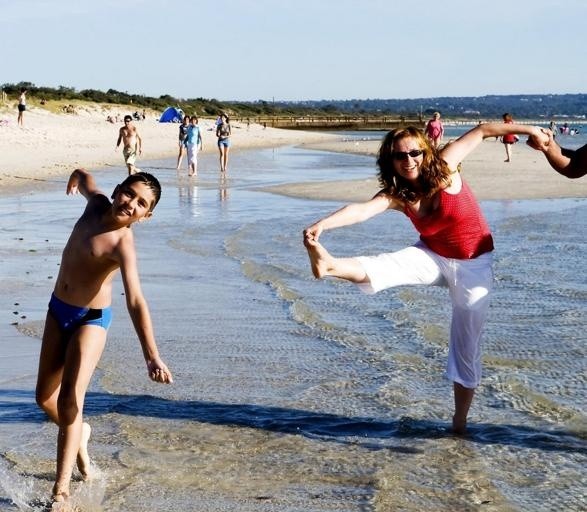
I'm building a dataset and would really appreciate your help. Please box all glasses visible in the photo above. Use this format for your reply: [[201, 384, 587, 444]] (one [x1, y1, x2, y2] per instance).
[[391, 148, 423, 160]]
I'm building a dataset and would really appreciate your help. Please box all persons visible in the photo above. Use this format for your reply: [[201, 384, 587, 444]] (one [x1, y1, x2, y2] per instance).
[[423, 112, 445, 147], [500, 112, 519, 163], [38, 98, 250, 177], [528, 126, 587, 180], [17, 87, 27, 126], [476, 120, 580, 143], [302, 126, 551, 435], [34, 169, 175, 505]]
